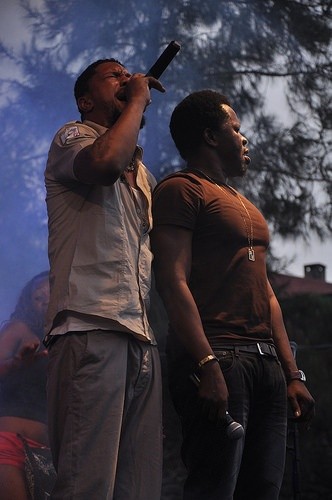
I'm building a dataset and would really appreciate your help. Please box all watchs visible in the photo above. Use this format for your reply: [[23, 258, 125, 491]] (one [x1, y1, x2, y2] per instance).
[[289, 369, 306, 381]]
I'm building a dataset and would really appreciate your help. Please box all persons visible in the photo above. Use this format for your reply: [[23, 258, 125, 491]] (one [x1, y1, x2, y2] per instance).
[[45, 58, 163, 500], [0, 270, 50, 500], [152, 91, 317, 500]]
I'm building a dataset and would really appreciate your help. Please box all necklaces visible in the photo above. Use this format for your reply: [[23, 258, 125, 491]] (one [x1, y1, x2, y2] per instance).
[[122, 162, 148, 236], [194, 168, 255, 262]]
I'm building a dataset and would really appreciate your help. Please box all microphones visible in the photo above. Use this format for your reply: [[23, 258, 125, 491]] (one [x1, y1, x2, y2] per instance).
[[144, 40, 181, 89], [191, 373, 244, 439]]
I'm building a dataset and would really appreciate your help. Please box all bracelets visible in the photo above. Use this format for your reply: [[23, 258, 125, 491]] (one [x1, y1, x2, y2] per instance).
[[195, 355, 220, 372]]
